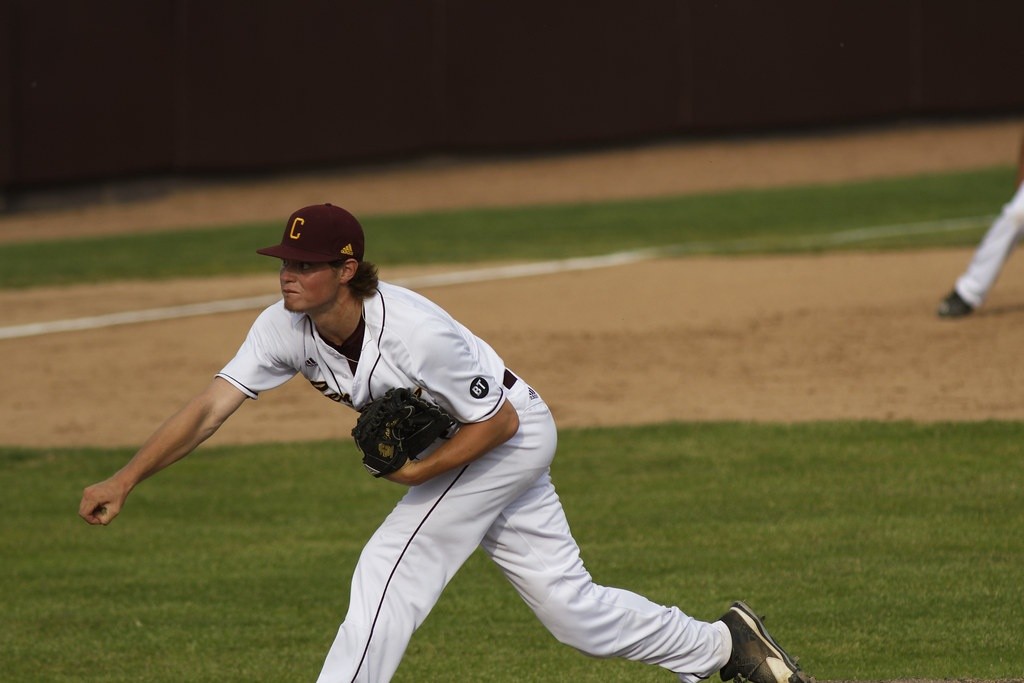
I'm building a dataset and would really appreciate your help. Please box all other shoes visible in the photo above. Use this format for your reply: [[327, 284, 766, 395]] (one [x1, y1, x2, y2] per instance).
[[939, 291, 969, 315]]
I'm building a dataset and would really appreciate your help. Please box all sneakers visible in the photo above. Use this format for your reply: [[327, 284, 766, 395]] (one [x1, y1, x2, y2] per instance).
[[720, 600, 814, 683]]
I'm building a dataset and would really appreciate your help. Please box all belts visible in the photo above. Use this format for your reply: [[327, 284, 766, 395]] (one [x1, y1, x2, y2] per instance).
[[503, 370, 517, 389]]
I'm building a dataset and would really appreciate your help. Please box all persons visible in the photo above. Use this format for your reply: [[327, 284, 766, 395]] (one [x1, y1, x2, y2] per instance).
[[937, 137, 1024, 318], [80, 203, 813, 683]]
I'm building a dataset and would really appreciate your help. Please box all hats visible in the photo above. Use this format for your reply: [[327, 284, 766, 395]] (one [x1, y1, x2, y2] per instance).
[[257, 203, 364, 263]]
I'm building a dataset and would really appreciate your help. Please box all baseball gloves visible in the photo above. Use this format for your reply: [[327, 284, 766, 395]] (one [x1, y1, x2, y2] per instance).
[[351, 388, 453, 476]]
